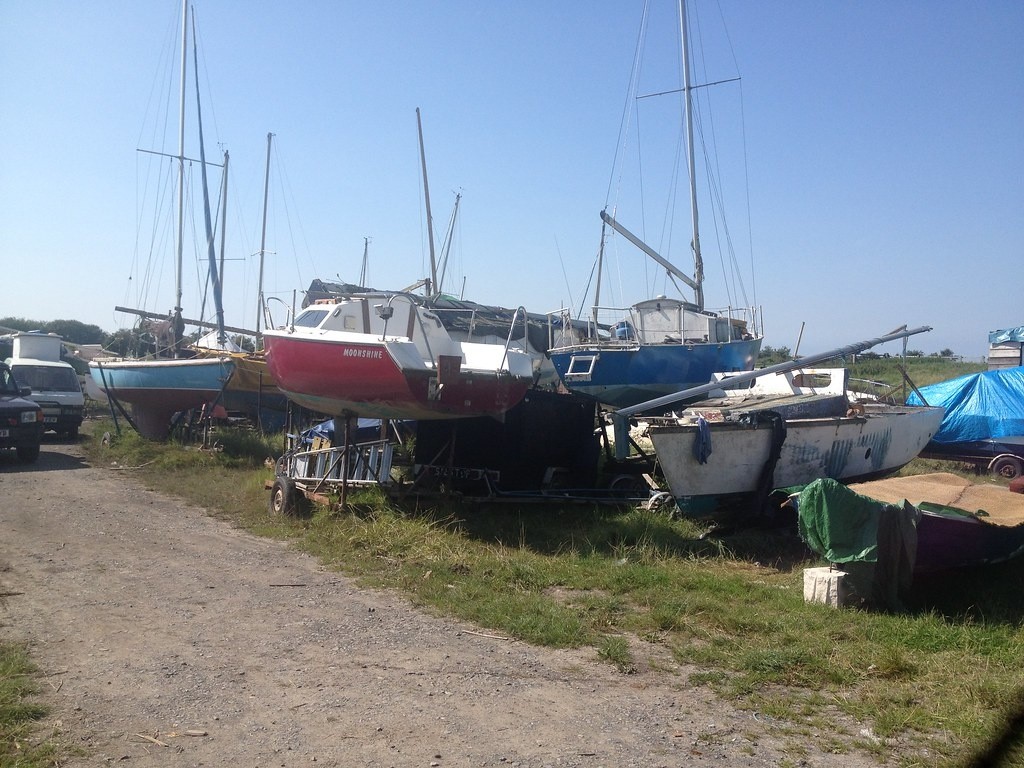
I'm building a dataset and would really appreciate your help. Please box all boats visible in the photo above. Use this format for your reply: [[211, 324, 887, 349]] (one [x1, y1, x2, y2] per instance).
[[646, 367, 947, 517], [259, 289, 536, 424]]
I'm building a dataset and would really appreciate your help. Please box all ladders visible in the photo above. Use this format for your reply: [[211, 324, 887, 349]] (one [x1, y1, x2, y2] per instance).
[[274, 434, 398, 492]]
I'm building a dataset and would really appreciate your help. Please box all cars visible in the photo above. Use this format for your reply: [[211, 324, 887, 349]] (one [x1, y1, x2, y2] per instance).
[[6, 356, 86, 439], [0, 361, 43, 464], [905, 365, 1024, 480]]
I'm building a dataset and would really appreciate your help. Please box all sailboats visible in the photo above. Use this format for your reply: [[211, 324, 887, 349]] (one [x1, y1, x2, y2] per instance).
[[542, 2, 768, 407], [191, 131, 289, 421], [88, 0, 233, 411]]
